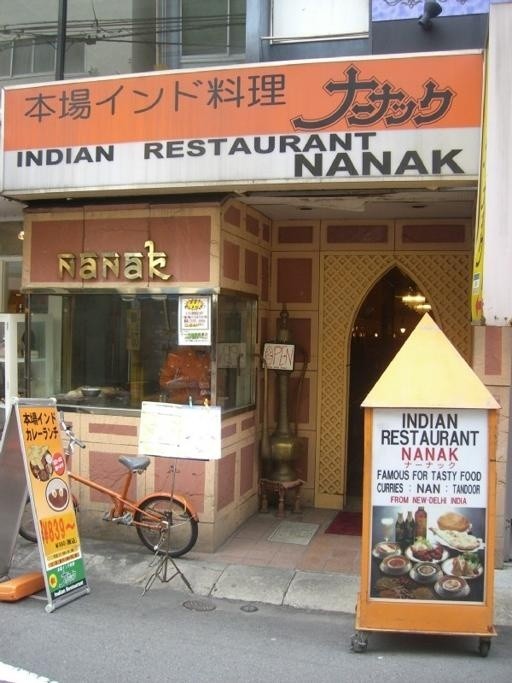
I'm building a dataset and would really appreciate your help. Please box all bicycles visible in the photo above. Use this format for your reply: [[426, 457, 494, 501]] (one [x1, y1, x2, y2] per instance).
[[19, 409, 199, 558]]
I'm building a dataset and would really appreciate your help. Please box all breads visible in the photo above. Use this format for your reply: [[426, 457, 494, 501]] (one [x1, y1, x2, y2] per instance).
[[437, 513, 468, 531]]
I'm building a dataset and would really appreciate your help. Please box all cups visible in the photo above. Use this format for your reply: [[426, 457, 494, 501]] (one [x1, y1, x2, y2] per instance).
[[380, 518, 393, 539], [32, 349, 38, 358]]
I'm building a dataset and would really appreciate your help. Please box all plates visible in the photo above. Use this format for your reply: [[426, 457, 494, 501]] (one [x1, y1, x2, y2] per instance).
[[408, 567, 444, 584], [441, 558, 483, 580], [433, 581, 470, 599], [379, 561, 412, 576], [436, 535, 482, 552], [372, 548, 401, 559], [405, 543, 448, 562]]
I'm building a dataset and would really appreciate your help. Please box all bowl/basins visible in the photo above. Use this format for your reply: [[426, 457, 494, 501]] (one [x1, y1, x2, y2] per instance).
[[415, 563, 440, 578], [438, 576, 465, 592], [81, 388, 101, 397], [384, 555, 410, 570], [376, 541, 399, 555]]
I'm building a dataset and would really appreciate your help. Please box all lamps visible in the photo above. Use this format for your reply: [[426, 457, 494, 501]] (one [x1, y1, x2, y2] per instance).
[[417, 1, 442, 30]]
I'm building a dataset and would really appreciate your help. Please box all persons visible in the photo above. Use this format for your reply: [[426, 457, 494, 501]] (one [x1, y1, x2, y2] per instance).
[[158, 344, 227, 404]]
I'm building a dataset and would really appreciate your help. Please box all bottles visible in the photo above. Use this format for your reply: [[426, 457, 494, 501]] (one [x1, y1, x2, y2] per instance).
[[414, 506, 427, 541], [395, 512, 405, 542], [405, 510, 414, 543]]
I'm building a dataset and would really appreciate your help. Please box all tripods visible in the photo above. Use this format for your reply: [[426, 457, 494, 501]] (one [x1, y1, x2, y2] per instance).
[[138, 471, 191, 594]]
